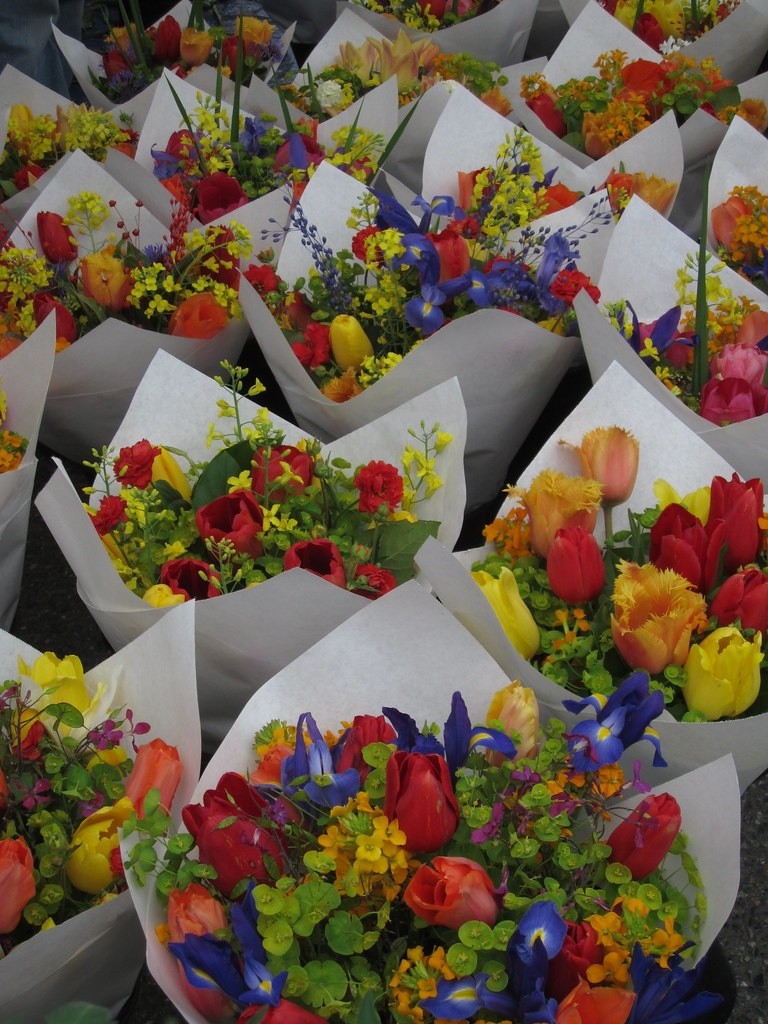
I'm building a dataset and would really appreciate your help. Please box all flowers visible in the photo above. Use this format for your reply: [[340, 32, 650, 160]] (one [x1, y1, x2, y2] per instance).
[[0, 0, 768, 1024]]
